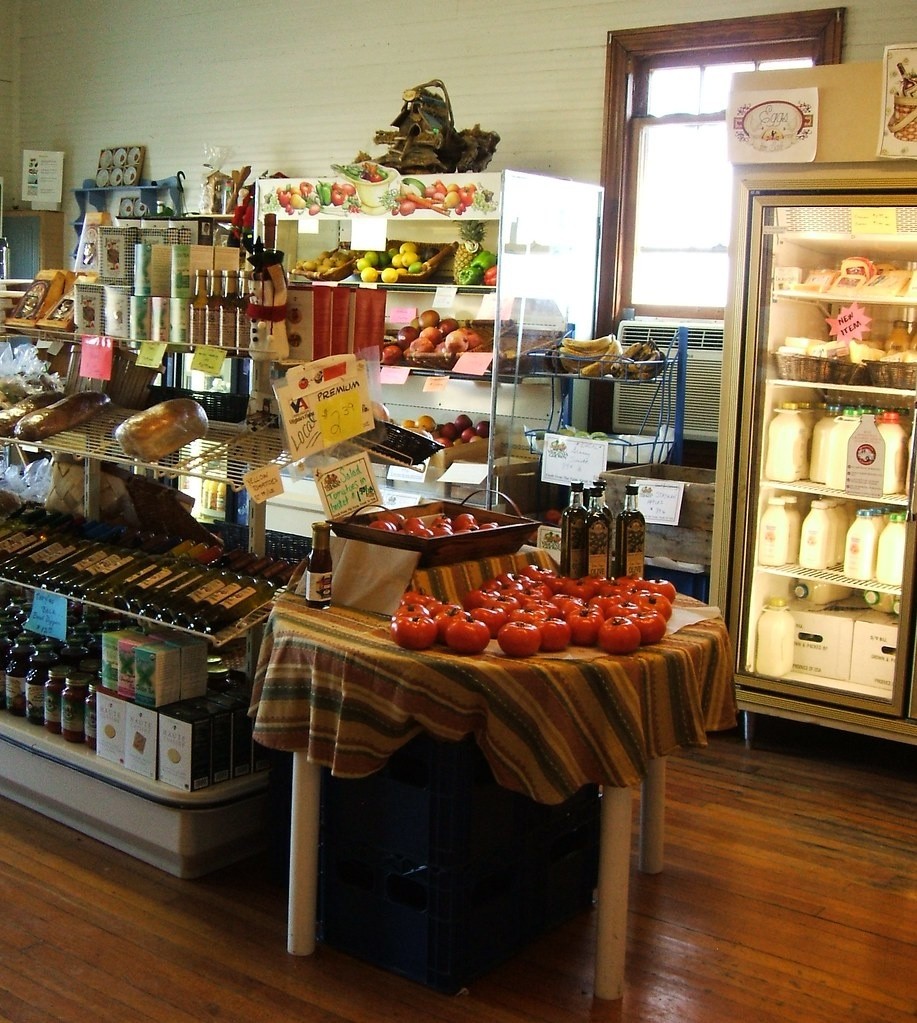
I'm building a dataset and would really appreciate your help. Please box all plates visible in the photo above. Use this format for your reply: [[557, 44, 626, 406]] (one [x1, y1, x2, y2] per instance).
[[96, 169, 108, 188], [100, 150, 112, 168], [124, 167, 137, 184], [109, 168, 123, 186], [128, 147, 141, 164], [114, 148, 127, 166], [120, 199, 133, 217], [134, 199, 146, 217]]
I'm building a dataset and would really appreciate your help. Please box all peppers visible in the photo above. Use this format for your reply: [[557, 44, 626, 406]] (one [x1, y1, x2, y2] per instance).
[[459, 250, 497, 286]]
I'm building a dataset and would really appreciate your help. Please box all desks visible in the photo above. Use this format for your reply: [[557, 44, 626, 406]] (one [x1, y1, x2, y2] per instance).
[[247, 586, 738, 1001]]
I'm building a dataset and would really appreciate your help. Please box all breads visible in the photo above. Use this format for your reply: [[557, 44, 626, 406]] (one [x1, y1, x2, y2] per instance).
[[115, 396, 209, 461], [0, 389, 114, 442]]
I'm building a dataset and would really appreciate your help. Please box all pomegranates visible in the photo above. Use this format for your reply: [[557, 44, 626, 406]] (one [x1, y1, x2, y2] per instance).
[[381, 311, 480, 368]]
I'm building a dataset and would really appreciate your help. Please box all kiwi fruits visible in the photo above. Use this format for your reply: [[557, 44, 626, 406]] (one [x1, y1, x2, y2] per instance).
[[294, 249, 356, 272]]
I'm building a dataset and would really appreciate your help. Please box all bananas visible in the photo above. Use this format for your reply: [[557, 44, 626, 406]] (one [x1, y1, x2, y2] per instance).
[[557, 333, 667, 380]]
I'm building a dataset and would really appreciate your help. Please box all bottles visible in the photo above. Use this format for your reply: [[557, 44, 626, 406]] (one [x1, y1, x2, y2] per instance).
[[615, 483, 645, 581], [157, 201, 165, 213], [885, 320, 910, 354], [561, 482, 588, 580], [0, 503, 300, 634], [222, 180, 233, 215], [756, 495, 907, 677], [909, 321, 917, 352], [766, 403, 917, 495], [593, 478, 613, 522], [190, 270, 250, 356], [583, 486, 612, 579], [306, 522, 332, 609]]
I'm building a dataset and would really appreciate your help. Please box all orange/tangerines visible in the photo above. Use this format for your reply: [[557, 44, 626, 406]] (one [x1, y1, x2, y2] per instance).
[[399, 414, 435, 436]]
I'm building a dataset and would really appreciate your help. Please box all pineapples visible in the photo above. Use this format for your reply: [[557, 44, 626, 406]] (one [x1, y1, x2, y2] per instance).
[[453, 219, 487, 284]]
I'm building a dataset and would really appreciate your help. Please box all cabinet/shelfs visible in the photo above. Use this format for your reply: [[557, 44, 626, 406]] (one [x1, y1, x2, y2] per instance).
[[68, 176, 181, 260], [526, 323, 686, 469], [251, 170, 606, 538]]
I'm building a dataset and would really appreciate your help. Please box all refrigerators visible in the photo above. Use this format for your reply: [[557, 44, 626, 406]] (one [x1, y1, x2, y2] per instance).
[[709, 61, 917, 751]]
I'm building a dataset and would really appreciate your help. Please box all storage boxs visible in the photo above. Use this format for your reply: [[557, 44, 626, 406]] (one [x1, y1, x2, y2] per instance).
[[849, 609, 900, 690], [435, 443, 542, 506], [0, 317, 427, 875], [65, 345, 167, 410], [101, 627, 207, 708], [787, 595, 870, 682], [285, 285, 387, 361], [598, 464, 716, 565], [96, 145, 146, 185], [94, 668, 265, 793], [386, 432, 508, 498]]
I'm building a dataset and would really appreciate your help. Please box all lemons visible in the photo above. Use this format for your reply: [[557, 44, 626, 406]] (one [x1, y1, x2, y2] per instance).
[[356, 242, 423, 284]]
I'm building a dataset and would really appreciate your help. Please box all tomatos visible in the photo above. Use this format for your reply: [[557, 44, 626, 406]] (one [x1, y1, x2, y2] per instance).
[[388, 565, 676, 658], [367, 510, 501, 538]]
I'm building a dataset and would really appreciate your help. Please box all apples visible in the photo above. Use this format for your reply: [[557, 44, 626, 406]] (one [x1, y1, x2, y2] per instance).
[[433, 414, 490, 448]]
